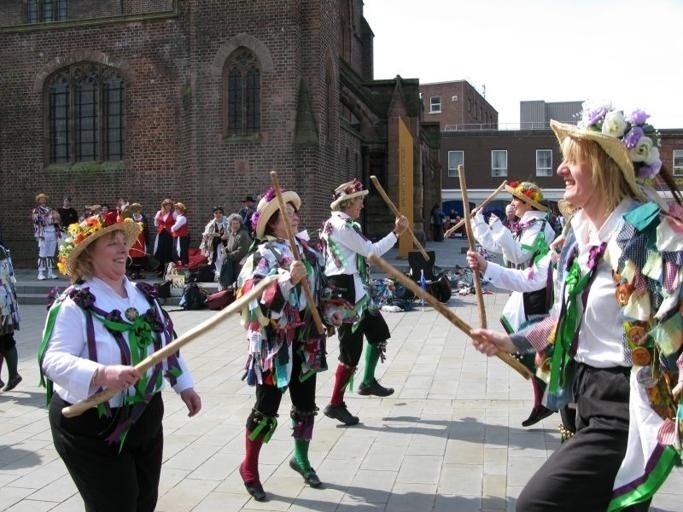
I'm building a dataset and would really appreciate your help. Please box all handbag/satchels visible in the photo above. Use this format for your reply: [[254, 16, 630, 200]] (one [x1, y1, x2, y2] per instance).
[[153, 280, 173, 298], [522, 290, 548, 317]]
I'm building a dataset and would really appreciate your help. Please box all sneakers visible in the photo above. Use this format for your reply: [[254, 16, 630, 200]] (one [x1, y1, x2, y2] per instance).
[[0, 374, 22, 392], [36, 270, 59, 280]]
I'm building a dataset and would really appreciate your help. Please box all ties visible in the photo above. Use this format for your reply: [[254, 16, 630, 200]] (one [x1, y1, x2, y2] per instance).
[[568, 242, 607, 357]]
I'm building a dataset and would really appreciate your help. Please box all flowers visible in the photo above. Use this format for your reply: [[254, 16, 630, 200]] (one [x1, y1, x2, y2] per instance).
[[510, 178, 544, 206], [249, 186, 277, 230], [332, 177, 362, 200], [580, 103, 664, 185]]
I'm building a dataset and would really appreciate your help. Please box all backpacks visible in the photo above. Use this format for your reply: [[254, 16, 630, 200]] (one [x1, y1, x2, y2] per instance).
[[178, 280, 204, 311]]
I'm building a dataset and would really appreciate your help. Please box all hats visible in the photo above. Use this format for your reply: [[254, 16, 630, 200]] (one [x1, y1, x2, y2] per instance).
[[548, 104, 662, 204], [329, 181, 369, 211], [35, 194, 257, 215], [504, 180, 547, 212], [249, 184, 302, 241]]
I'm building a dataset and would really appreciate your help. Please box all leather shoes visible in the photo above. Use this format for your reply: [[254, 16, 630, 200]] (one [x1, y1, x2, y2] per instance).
[[357, 380, 395, 397], [289, 456, 321, 487], [322, 401, 359, 425], [520, 404, 554, 426], [239, 462, 268, 503]]
